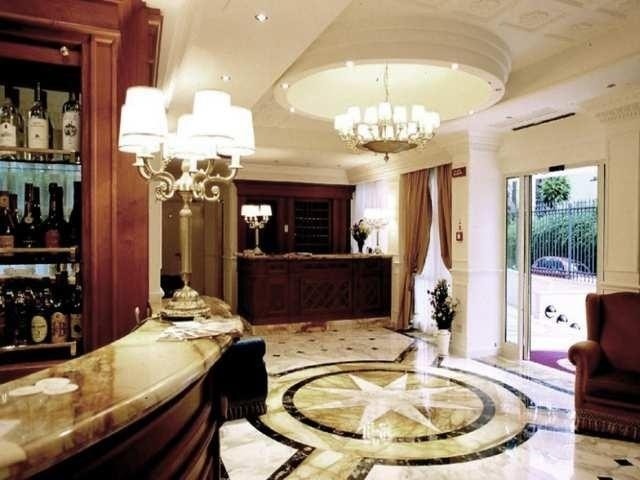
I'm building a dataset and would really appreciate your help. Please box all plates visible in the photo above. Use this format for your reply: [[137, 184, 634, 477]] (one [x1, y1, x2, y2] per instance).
[[9, 377, 80, 398]]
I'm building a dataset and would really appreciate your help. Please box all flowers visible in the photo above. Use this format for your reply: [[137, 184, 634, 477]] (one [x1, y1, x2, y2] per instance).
[[427, 279, 459, 329], [353, 220, 370, 240]]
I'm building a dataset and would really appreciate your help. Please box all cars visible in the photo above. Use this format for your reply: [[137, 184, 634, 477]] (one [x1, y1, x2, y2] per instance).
[[532, 256, 590, 279]]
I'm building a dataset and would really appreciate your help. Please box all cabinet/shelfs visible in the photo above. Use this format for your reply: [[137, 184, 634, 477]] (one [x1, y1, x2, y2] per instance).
[[0, 31, 121, 382]]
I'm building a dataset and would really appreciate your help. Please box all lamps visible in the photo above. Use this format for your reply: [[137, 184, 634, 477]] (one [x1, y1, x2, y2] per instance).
[[365, 213, 390, 253], [240, 202, 272, 255], [116, 86, 258, 321], [332, 67, 444, 160]]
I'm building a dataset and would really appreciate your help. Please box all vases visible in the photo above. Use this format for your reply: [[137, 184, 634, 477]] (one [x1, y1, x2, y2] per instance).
[[357, 239, 364, 252], [436, 329, 451, 357]]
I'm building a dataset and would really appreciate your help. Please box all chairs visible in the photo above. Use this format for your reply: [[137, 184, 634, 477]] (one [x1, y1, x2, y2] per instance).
[[222, 337, 271, 420], [567, 291, 639, 443]]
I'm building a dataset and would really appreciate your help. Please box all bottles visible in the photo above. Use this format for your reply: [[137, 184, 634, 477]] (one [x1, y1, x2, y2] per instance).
[[0, 78, 81, 165], [0, 181, 82, 250], [0, 270, 83, 346]]
